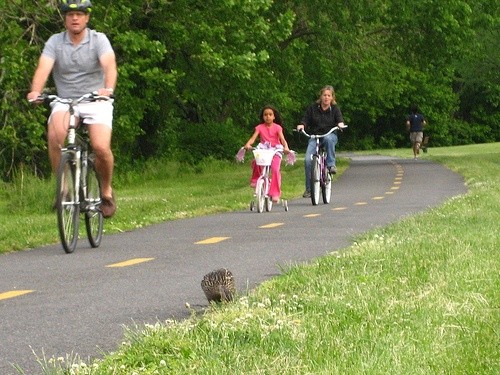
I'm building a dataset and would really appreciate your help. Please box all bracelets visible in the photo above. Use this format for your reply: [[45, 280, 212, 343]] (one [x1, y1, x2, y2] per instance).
[[105, 88, 114, 91]]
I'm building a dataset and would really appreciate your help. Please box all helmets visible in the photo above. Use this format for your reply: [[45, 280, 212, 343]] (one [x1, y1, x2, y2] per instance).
[[60, 0, 94, 15]]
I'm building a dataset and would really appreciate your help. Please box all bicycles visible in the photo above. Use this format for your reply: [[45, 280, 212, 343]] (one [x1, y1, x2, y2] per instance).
[[24, 89, 115, 253], [235, 145, 298, 213], [294, 125, 349, 205]]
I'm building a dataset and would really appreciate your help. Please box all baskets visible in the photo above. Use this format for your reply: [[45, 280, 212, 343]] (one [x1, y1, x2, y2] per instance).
[[253, 148, 275, 167]]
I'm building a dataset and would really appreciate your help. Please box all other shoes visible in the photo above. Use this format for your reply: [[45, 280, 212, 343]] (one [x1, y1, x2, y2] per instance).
[[51, 193, 71, 213], [330, 167, 336, 174], [303, 191, 312, 198], [250, 182, 256, 188], [99, 187, 116, 218], [271, 195, 280, 204]]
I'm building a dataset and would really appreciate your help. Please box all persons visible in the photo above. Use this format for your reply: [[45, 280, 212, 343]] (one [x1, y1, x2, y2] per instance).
[[244, 106, 289, 204], [406, 105, 426, 160], [26, 0, 117, 219], [297, 85, 344, 198]]
